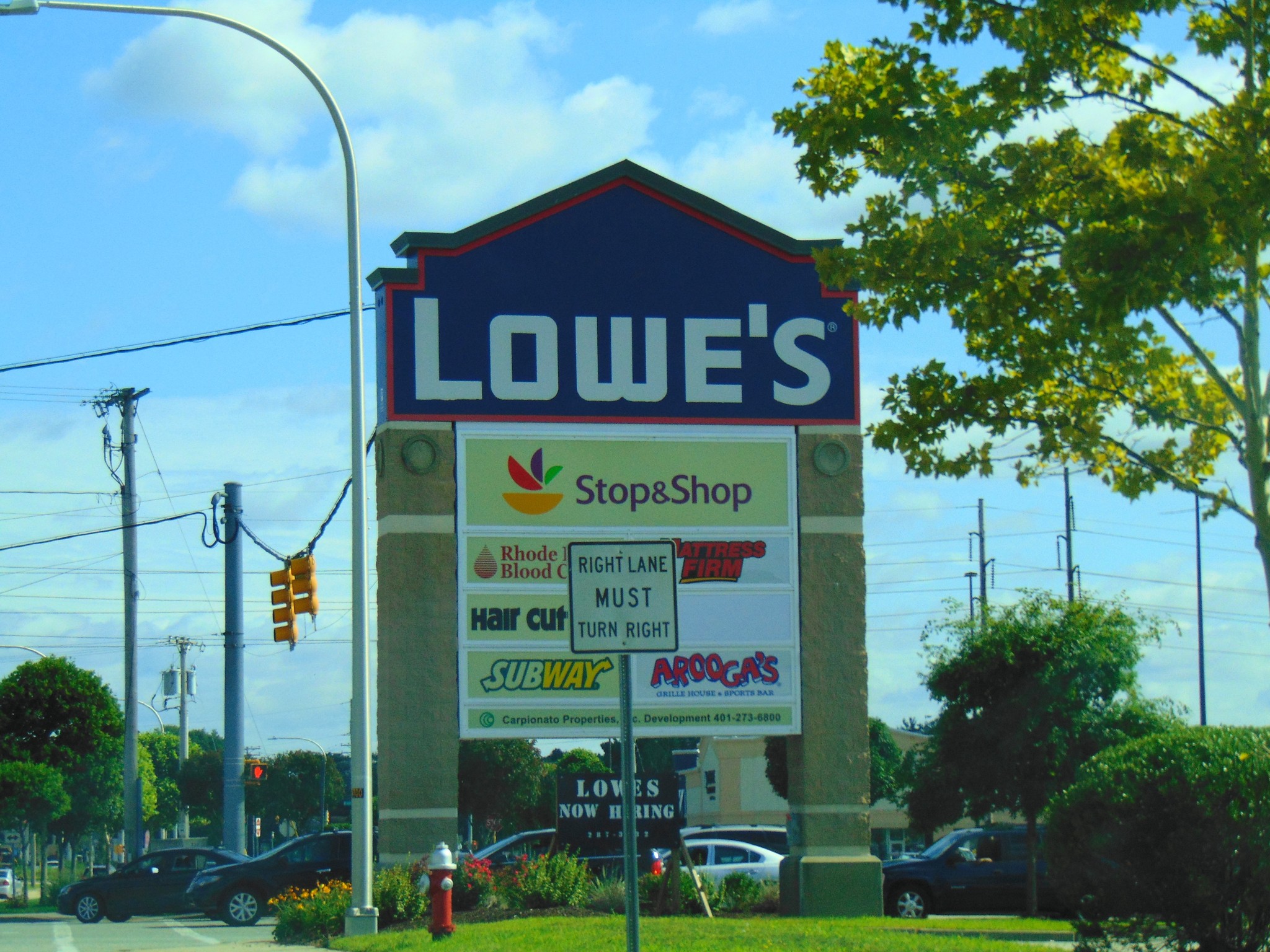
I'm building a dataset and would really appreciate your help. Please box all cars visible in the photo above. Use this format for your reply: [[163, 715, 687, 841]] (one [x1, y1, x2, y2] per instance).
[[57, 847, 255, 923], [660, 839, 789, 887], [0, 868, 23, 899]]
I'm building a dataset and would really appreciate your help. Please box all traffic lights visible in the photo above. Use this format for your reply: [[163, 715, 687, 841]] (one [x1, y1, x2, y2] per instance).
[[289, 555, 320, 616], [248, 762, 269, 782], [268, 568, 296, 653]]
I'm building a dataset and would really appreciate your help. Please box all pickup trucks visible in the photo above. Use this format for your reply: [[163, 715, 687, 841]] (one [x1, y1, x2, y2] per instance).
[[462, 829, 672, 913]]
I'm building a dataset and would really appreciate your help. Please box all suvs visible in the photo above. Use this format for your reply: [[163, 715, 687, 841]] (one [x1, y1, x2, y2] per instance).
[[189, 827, 380, 926], [679, 823, 789, 859]]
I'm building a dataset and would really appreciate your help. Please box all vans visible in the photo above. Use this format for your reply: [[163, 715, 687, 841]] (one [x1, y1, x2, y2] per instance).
[[881, 823, 1100, 916]]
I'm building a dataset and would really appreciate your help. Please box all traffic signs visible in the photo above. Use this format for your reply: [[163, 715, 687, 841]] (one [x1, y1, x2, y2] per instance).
[[563, 536, 686, 656]]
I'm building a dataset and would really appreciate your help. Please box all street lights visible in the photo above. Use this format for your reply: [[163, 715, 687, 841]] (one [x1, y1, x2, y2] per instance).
[[0, 1, 386, 945], [267, 736, 327, 832]]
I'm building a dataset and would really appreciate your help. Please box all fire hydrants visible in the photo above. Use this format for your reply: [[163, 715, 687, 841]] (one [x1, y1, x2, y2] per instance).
[[418, 839, 458, 945]]
